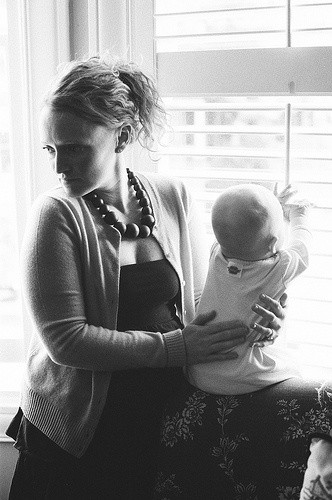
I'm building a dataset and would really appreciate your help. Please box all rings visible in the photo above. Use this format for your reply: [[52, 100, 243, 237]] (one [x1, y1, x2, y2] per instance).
[[268, 330, 276, 340]]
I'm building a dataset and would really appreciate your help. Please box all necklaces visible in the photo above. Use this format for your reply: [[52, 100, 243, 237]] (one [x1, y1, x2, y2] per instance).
[[84, 167, 155, 238]]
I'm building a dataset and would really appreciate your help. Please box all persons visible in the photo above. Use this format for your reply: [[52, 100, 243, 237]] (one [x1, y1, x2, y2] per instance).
[[5, 53, 332, 500], [182, 180, 315, 396]]
